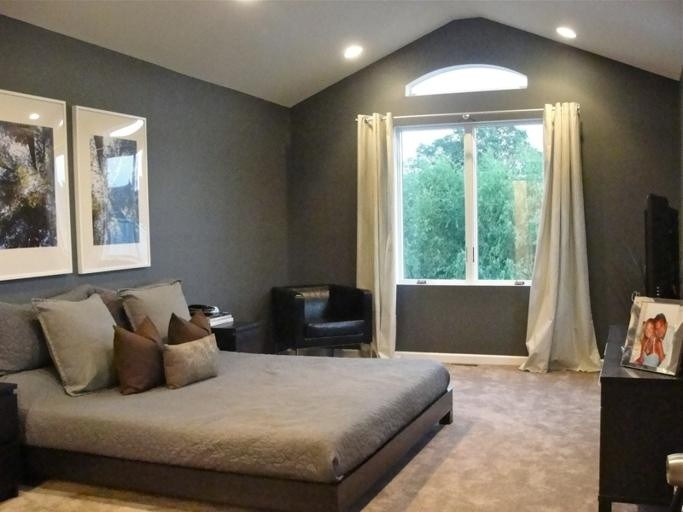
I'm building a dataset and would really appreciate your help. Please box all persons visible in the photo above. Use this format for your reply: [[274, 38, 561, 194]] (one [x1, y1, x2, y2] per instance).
[[655, 313, 676, 368], [634, 318, 664, 368]]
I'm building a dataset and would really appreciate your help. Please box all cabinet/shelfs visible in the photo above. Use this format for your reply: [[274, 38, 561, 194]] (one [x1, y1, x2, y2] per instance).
[[597, 322, 683, 512]]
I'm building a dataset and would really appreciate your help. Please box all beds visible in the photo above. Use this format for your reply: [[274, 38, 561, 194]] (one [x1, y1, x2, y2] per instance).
[[0, 274, 454, 510]]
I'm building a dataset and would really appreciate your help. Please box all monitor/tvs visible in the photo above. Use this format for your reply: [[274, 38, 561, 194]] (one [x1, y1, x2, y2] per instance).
[[644, 194, 681, 299]]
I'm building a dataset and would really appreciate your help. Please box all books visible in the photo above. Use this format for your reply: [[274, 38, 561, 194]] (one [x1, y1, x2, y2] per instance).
[[207, 311, 234, 327]]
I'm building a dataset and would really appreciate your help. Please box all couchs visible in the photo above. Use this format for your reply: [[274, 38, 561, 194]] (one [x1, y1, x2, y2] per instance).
[[269, 282, 373, 355]]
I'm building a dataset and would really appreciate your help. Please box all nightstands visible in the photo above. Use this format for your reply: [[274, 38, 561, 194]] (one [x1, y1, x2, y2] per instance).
[[0, 379, 19, 503], [209, 319, 265, 354]]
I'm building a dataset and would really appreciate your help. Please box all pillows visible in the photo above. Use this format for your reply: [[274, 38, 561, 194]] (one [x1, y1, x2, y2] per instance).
[[166, 309, 212, 344], [118, 279, 191, 337], [109, 315, 162, 395], [0, 283, 91, 378], [161, 332, 219, 389], [29, 291, 117, 398], [88, 279, 183, 332]]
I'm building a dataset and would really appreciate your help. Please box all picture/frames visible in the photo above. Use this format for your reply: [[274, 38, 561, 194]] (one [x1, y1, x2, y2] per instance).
[[617, 296, 681, 378], [71, 105, 152, 274], [0, 91, 72, 282]]
